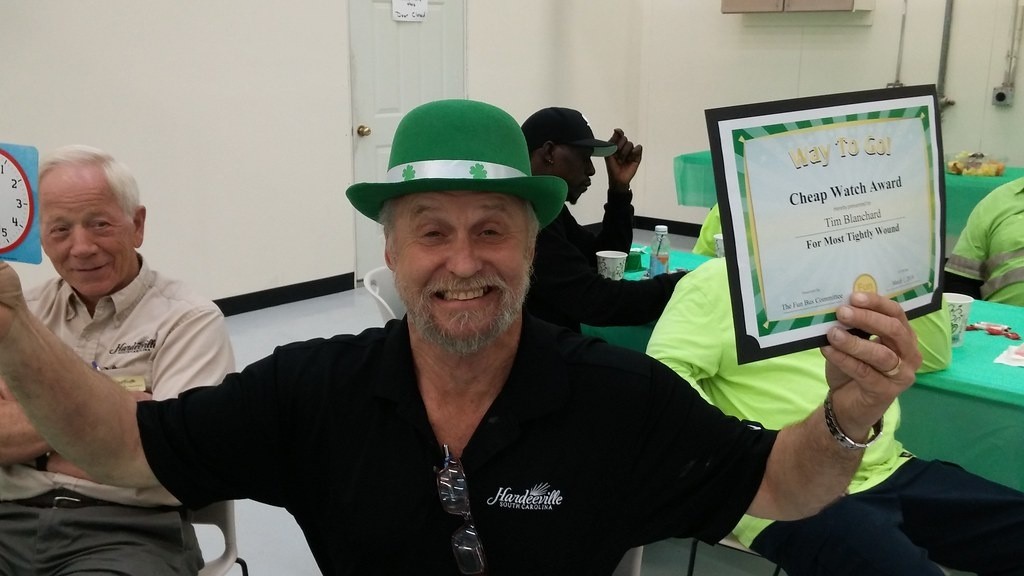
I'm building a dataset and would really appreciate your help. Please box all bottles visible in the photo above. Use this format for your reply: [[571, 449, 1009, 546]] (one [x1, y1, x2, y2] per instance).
[[649, 225, 670, 279]]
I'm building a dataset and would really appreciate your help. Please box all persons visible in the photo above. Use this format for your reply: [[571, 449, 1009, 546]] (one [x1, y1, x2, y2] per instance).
[[943, 176, 1024, 307], [0, 145, 237, 576], [0, 97, 924, 576], [645, 256, 1024, 576], [692, 202, 723, 257], [517, 106, 693, 335]]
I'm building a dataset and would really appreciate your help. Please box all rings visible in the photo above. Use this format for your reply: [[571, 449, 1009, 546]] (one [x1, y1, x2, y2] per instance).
[[885, 356, 901, 375]]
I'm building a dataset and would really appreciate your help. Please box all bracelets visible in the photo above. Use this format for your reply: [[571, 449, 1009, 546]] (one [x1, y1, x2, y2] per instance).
[[823, 389, 884, 450], [36, 452, 50, 471]]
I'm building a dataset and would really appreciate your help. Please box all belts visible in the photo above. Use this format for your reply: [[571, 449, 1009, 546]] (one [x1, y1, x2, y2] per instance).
[[4, 488, 119, 509]]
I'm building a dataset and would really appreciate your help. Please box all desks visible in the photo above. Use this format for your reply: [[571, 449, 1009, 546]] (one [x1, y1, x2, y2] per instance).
[[675, 149, 1024, 227], [581, 238, 1023, 496]]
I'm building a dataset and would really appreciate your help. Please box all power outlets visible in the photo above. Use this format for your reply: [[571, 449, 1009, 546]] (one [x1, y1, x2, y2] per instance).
[[993, 87, 1015, 107]]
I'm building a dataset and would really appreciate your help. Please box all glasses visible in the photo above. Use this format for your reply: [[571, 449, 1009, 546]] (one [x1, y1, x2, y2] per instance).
[[435, 443, 486, 576]]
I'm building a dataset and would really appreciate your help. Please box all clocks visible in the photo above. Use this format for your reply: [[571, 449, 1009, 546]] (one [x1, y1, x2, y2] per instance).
[[0, 142, 43, 265]]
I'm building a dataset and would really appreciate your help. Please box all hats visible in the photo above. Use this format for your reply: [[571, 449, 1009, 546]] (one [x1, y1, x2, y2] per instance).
[[522, 107, 619, 158], [346, 99, 572, 232]]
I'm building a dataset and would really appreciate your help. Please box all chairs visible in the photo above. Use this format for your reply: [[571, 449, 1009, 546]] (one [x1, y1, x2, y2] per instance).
[[193, 496, 249, 576], [688, 532, 781, 576], [364, 265, 406, 325]]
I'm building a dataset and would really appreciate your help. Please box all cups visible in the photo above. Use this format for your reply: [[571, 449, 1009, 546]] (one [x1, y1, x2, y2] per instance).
[[942, 292, 975, 348], [713, 234, 725, 258], [596, 251, 628, 280]]
